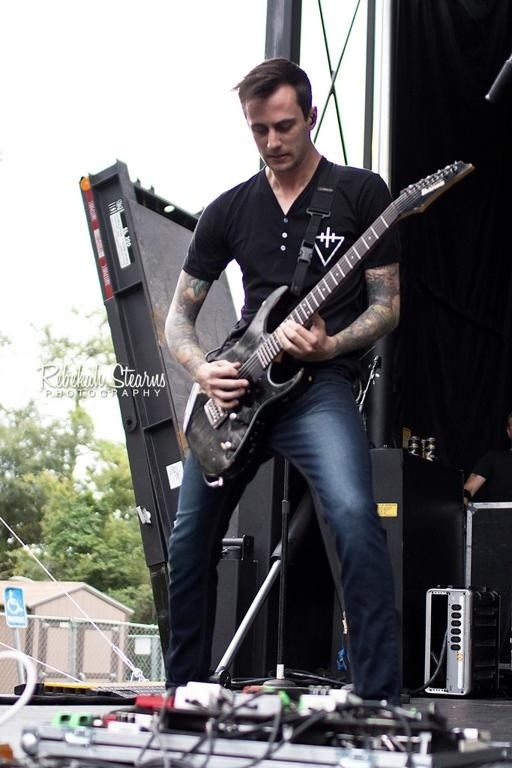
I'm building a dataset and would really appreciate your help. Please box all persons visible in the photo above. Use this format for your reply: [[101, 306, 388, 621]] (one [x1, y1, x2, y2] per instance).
[[459, 413, 510, 575], [158, 58, 406, 713]]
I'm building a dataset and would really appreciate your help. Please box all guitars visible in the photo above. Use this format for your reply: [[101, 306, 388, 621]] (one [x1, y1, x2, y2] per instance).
[[182, 160, 475, 482]]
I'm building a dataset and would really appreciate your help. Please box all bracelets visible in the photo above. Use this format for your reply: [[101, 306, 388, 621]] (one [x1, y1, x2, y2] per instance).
[[462, 486, 471, 501]]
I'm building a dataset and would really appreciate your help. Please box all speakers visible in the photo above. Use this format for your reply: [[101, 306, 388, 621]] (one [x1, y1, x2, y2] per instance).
[[330, 447, 464, 694]]
